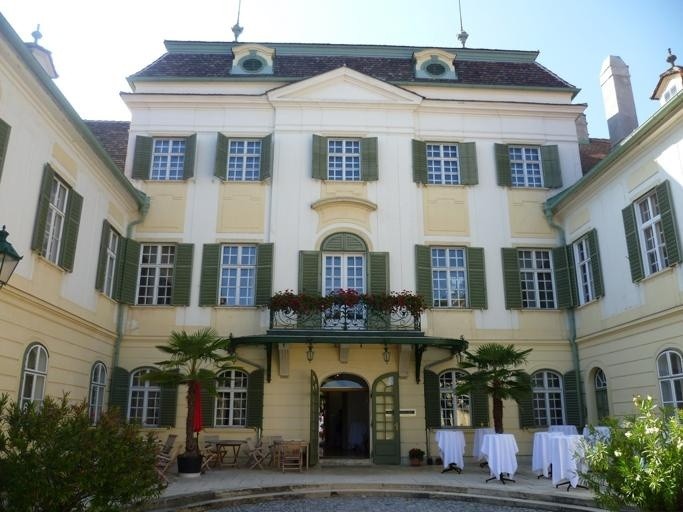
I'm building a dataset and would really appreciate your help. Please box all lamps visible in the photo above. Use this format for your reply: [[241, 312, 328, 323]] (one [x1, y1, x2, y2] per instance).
[[0, 225, 24, 289], [306, 346, 314, 361], [382, 348, 391, 362]]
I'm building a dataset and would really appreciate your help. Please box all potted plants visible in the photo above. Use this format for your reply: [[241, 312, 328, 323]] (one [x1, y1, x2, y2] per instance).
[[409, 448, 425, 465]]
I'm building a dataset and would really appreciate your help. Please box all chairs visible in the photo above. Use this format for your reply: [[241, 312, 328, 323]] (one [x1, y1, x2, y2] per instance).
[[154, 434, 303, 483]]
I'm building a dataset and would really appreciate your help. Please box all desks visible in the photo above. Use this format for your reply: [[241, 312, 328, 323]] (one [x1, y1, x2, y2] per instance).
[[434, 425, 610, 493], [278, 441, 309, 469]]
